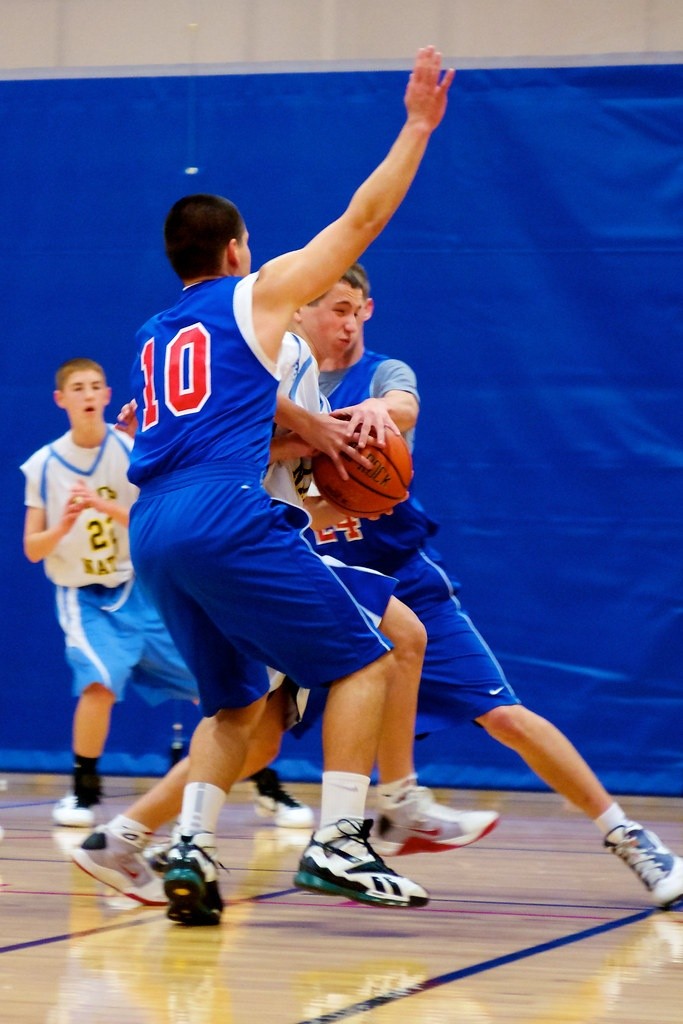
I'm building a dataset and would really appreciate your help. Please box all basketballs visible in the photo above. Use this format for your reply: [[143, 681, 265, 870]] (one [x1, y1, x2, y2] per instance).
[[313, 419, 415, 518]]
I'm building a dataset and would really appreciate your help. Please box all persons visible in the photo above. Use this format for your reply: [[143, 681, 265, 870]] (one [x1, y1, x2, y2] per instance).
[[125, 45, 456, 925], [315, 262, 682, 908], [71, 268, 500, 904], [21, 360, 313, 829]]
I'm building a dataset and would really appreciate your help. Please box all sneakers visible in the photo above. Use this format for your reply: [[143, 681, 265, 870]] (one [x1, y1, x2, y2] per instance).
[[143, 832, 217, 871], [68, 824, 174, 906], [293, 818, 429, 908], [52, 796, 96, 826], [163, 836, 230, 925], [367, 789, 499, 857], [602, 821, 683, 908], [254, 791, 313, 828]]
